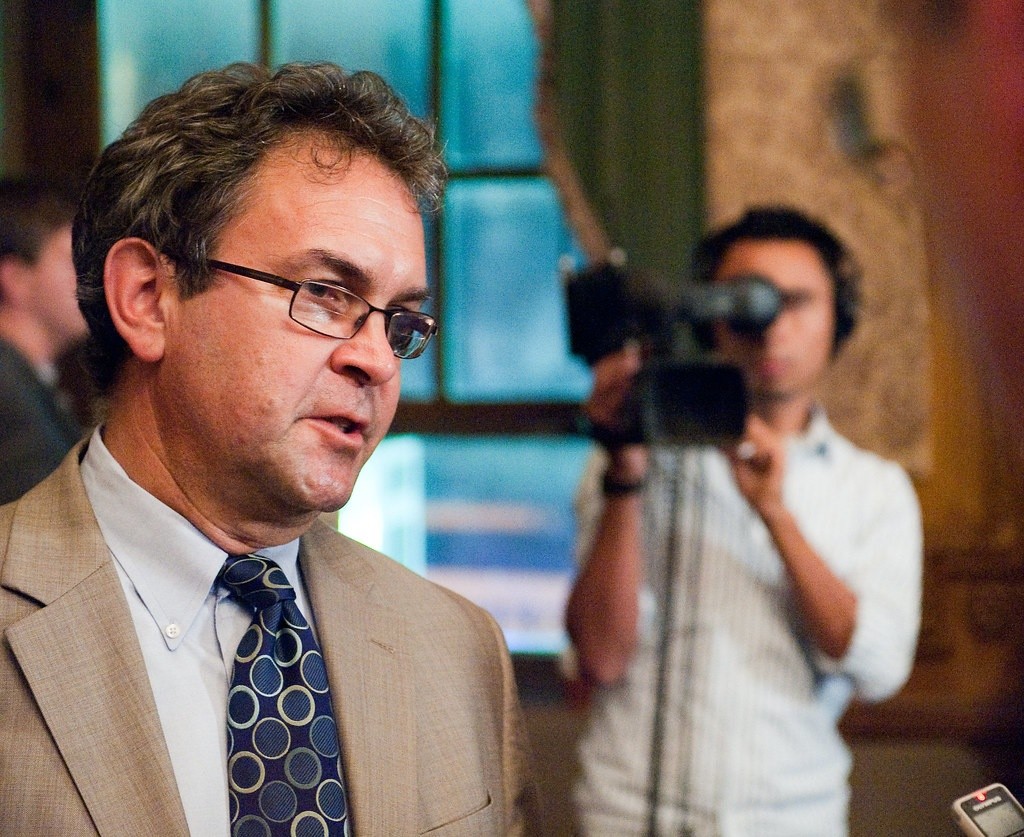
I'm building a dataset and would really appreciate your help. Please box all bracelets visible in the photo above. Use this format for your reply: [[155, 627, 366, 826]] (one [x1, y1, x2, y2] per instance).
[[600, 471, 646, 499]]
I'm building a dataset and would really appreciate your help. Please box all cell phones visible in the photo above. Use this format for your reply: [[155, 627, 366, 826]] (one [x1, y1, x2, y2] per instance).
[[948, 783, 1024, 837]]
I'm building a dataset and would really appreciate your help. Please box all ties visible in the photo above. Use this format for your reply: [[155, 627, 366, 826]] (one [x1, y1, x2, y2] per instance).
[[213, 554, 351, 837]]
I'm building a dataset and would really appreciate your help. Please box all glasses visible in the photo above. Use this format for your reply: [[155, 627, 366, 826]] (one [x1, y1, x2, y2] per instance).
[[204, 258, 437, 360]]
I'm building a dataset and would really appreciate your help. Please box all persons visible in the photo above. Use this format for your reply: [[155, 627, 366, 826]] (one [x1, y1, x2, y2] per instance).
[[0, 167, 88, 502], [0, 59, 543, 836], [567, 205, 926, 837]]
